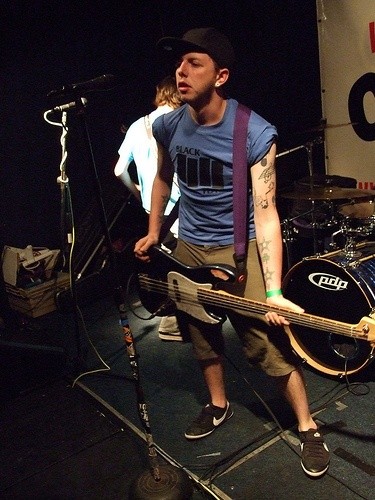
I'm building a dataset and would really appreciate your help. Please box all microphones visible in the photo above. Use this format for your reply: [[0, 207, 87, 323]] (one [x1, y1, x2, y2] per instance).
[[55, 97, 88, 110], [47, 74, 121, 98]]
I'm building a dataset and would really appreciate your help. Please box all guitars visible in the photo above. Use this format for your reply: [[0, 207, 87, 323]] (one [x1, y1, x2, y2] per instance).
[[133, 246, 375, 349]]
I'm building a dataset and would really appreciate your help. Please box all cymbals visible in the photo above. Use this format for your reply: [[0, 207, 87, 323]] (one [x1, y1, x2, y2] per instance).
[[277, 182, 375, 202]]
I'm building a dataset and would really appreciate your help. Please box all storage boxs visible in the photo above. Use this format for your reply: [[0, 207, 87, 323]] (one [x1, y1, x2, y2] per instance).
[[6, 270, 72, 319]]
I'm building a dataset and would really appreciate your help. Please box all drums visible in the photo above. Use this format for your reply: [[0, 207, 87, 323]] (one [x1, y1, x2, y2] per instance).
[[280, 244, 375, 381], [290, 208, 345, 256], [339, 202, 375, 238]]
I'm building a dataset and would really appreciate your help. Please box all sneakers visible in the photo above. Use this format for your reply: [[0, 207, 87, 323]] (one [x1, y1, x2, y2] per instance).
[[184, 399, 234, 439], [298, 425, 331, 476], [158, 331, 183, 341]]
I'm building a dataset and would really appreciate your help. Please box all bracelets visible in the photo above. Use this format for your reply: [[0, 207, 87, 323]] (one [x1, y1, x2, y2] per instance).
[[266, 290, 283, 297]]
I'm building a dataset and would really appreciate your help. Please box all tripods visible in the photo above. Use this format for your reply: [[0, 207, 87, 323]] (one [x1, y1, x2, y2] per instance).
[[17, 110, 135, 395]]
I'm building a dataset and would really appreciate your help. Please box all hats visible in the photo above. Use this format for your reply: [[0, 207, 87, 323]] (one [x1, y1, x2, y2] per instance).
[[156, 27, 235, 66]]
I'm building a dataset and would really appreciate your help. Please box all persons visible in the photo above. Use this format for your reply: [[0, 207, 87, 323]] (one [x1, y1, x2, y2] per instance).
[[134, 28, 331, 476], [114, 77, 183, 340]]
